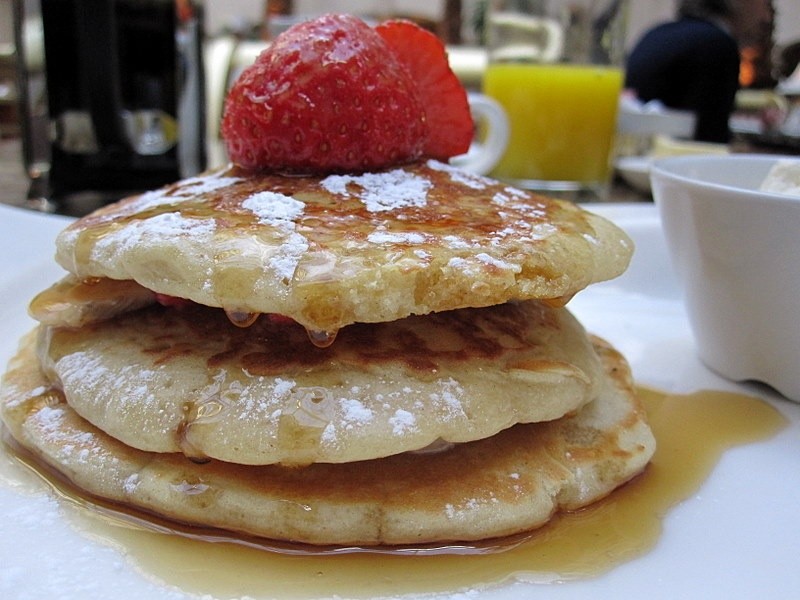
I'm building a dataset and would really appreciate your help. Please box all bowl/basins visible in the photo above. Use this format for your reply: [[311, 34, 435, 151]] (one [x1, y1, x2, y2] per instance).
[[647, 153, 799, 407]]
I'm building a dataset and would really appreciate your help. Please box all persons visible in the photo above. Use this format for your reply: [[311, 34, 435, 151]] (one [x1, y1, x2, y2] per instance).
[[617, 1, 763, 157]]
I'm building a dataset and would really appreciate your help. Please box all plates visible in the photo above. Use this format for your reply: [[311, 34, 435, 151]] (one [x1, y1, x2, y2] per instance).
[[614, 155, 649, 195]]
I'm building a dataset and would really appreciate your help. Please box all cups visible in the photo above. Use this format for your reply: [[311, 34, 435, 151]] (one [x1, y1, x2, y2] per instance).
[[479, 0, 630, 199]]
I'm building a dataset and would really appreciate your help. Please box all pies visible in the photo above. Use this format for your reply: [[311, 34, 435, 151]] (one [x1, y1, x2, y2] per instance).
[[14, 11, 664, 558]]
